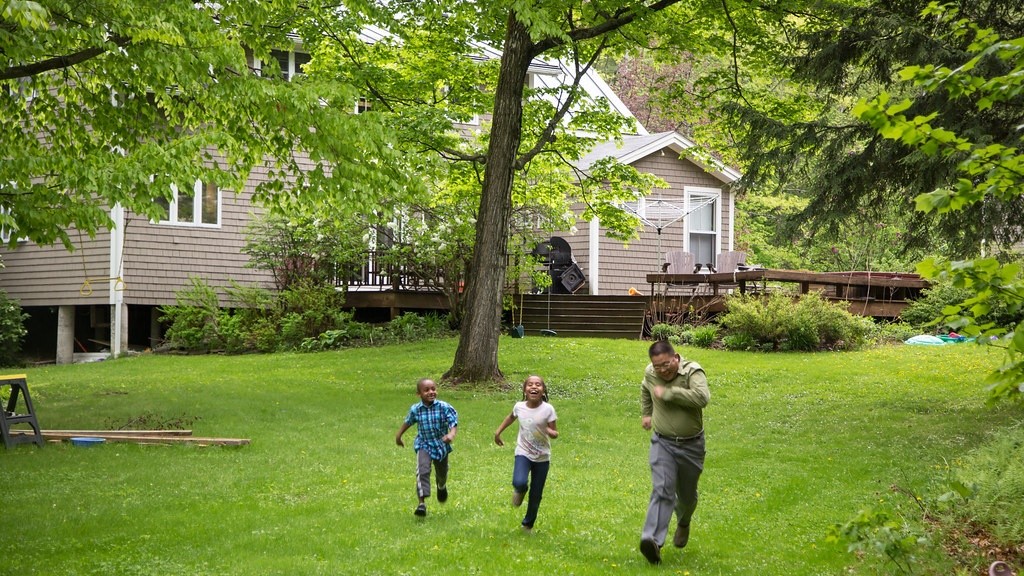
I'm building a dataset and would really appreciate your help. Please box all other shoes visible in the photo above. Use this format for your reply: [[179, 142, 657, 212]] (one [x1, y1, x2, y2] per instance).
[[414, 503, 426, 517], [673, 522, 690, 549], [512, 487, 528, 506], [436, 480, 448, 503], [520, 524, 531, 534], [640, 538, 662, 565]]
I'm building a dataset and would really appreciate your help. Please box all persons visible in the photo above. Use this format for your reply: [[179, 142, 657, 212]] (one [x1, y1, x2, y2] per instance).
[[494, 375, 558, 531], [639, 340, 710, 566], [396, 377, 458, 516]]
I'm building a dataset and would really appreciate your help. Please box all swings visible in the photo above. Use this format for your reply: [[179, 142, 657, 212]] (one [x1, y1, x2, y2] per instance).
[[510, 156, 529, 340], [538, 130, 558, 340]]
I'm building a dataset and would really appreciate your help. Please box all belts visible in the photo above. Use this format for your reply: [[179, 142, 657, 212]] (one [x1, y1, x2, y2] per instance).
[[653, 428, 704, 442]]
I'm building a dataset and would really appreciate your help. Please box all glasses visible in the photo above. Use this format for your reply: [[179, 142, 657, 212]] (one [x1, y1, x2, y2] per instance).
[[652, 353, 676, 371]]
